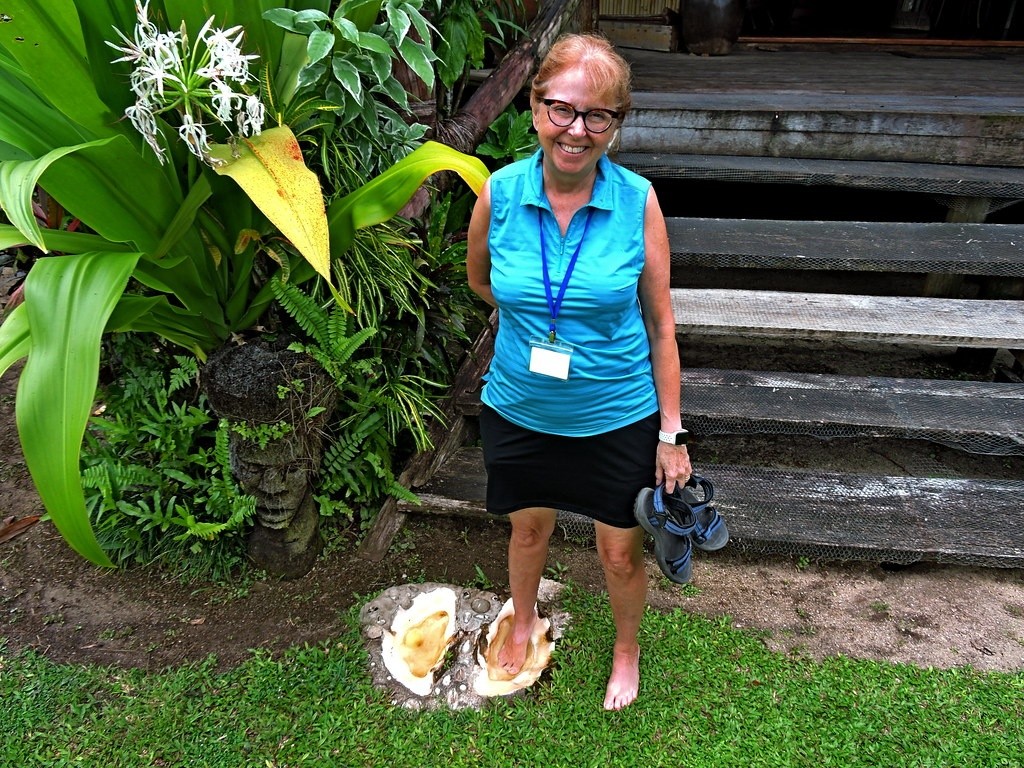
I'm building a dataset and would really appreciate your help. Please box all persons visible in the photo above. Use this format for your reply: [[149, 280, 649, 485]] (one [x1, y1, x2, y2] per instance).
[[466, 36, 692, 708]]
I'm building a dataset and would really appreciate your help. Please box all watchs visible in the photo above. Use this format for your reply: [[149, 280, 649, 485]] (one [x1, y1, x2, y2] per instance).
[[658, 429, 689, 445]]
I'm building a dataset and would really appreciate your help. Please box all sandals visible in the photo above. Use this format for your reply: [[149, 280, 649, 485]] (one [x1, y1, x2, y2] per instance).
[[655, 474, 729, 552], [633, 483, 694, 584]]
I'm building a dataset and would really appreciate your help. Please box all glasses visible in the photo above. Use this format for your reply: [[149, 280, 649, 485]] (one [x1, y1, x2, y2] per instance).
[[538, 96, 629, 135]]
